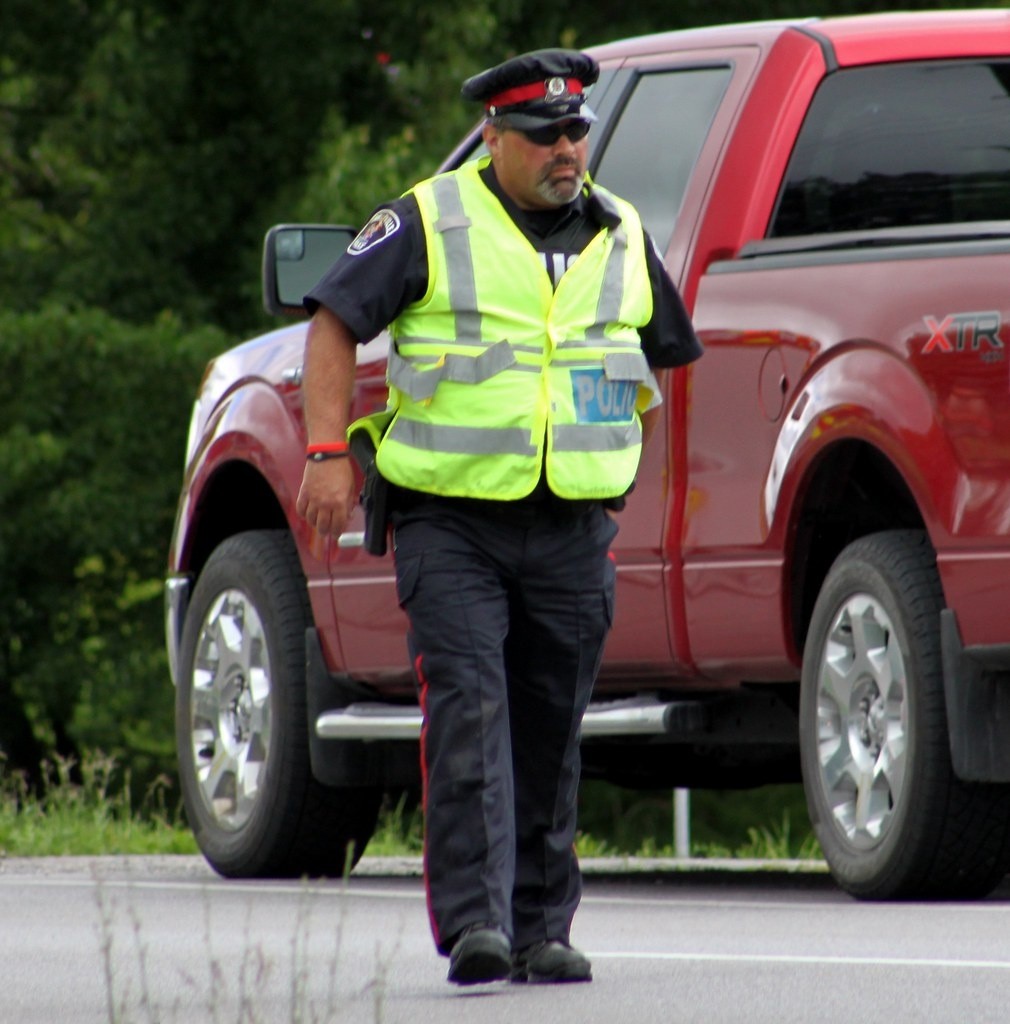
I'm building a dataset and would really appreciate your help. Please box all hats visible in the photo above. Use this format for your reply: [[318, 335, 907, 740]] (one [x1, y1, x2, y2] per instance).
[[459, 49, 599, 130]]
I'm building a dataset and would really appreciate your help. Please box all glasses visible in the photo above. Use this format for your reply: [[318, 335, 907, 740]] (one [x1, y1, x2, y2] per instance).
[[504, 117, 589, 146]]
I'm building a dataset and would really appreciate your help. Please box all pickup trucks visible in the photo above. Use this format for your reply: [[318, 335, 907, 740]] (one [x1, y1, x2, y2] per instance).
[[162, 6, 1010, 900]]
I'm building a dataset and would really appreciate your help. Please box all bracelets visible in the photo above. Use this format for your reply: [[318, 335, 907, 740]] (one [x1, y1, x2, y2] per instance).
[[306, 443, 349, 454]]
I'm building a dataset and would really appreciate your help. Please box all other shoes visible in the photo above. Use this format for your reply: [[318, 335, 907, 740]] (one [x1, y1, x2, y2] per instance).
[[505, 939, 590, 982], [448, 923, 510, 985]]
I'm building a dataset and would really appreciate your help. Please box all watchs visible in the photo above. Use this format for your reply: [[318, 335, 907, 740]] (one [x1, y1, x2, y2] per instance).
[[307, 452, 350, 461]]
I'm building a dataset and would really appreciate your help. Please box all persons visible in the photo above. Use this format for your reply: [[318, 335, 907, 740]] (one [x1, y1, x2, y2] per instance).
[[293, 46, 706, 985]]
